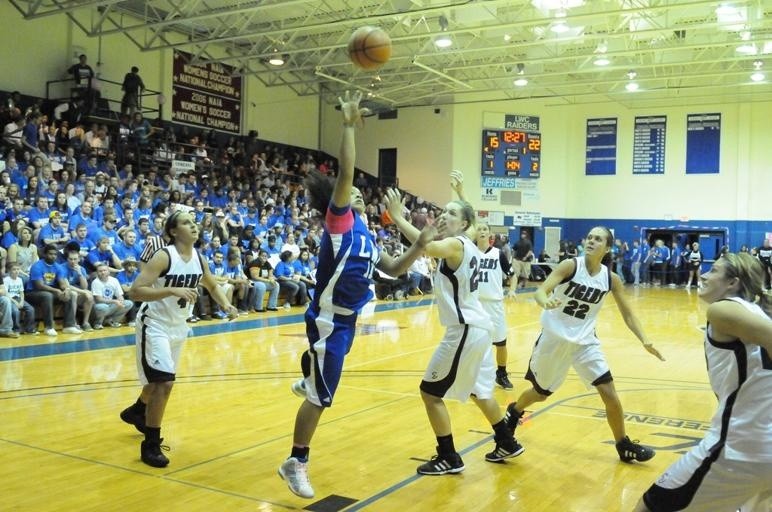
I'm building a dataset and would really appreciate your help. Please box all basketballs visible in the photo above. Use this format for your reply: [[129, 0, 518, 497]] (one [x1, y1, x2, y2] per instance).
[[350, 26, 392, 70]]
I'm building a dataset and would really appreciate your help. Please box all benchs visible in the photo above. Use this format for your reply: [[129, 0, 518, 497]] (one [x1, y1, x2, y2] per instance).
[[0, 289, 298, 332]]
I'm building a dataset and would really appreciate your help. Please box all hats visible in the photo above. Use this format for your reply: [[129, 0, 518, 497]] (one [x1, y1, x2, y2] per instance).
[[248, 208, 256, 213], [245, 225, 255, 230], [105, 214, 116, 222], [121, 258, 140, 268], [63, 161, 75, 166], [277, 207, 284, 211], [275, 222, 283, 227], [204, 208, 213, 213], [268, 236, 276, 243], [95, 170, 105, 177], [216, 211, 225, 217], [49, 211, 62, 223]]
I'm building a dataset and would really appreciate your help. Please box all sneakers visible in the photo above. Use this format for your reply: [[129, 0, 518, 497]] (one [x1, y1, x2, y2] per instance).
[[685, 285, 691, 288], [110, 322, 122, 327], [668, 283, 676, 287], [94, 324, 104, 329], [384, 294, 393, 301], [283, 302, 290, 308], [485, 437, 523, 462], [240, 309, 248, 314], [190, 317, 196, 323], [495, 370, 513, 390], [212, 310, 227, 319], [28, 329, 40, 335], [200, 315, 211, 320], [616, 436, 656, 463], [141, 438, 170, 468], [506, 402, 524, 436], [120, 403, 145, 433], [44, 328, 58, 336], [1, 331, 19, 338], [128, 323, 136, 327], [278, 456, 315, 498], [62, 327, 81, 334], [395, 290, 405, 301], [249, 309, 256, 313], [292, 380, 306, 398], [256, 309, 267, 312], [304, 302, 309, 308], [225, 306, 239, 317], [82, 323, 93, 331], [634, 282, 639, 285], [266, 308, 278, 311], [192, 316, 200, 320], [417, 452, 465, 475]]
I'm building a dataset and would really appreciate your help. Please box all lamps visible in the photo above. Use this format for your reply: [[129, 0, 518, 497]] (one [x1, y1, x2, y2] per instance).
[[269, 44, 285, 65], [513, 64, 530, 87], [157, 94, 166, 105], [435, 15, 453, 47]]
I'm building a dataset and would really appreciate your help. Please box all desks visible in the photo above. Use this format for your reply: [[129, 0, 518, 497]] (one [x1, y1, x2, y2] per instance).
[[531, 262, 559, 266]]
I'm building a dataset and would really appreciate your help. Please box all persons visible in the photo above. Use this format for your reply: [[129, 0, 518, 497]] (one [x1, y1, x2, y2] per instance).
[[502, 224, 666, 463], [718, 238, 771, 288], [385, 170, 524, 476], [632, 252, 772, 512], [120, 209, 239, 467], [67, 54, 94, 108], [473, 221, 519, 391], [1, 91, 445, 336], [120, 66, 146, 119], [278, 87, 450, 499], [490, 229, 704, 290]]
